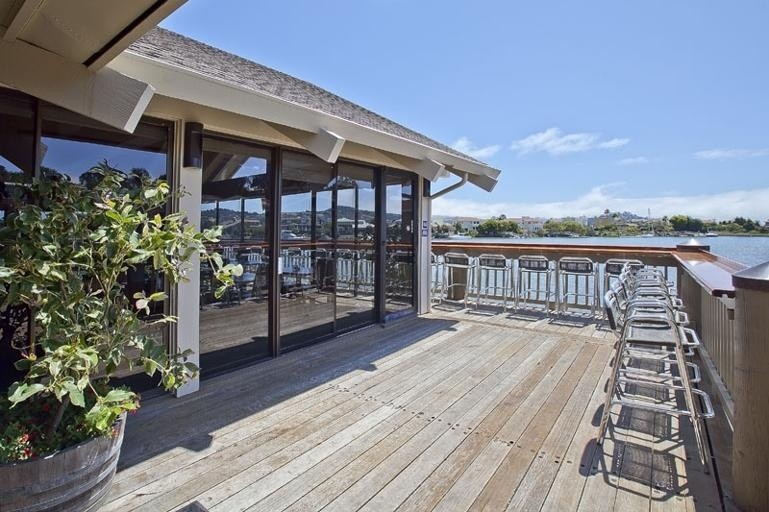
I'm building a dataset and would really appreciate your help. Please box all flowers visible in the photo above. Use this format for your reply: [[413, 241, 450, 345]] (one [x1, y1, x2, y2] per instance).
[[1, 399, 53, 463]]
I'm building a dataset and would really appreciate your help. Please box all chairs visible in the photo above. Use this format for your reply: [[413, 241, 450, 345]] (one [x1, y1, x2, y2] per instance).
[[216, 240, 412, 298], [434, 244, 595, 310], [593, 259, 719, 480]]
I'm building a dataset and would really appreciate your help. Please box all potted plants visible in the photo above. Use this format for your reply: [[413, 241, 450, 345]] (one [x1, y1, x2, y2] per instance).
[[0, 164, 250, 512]]
